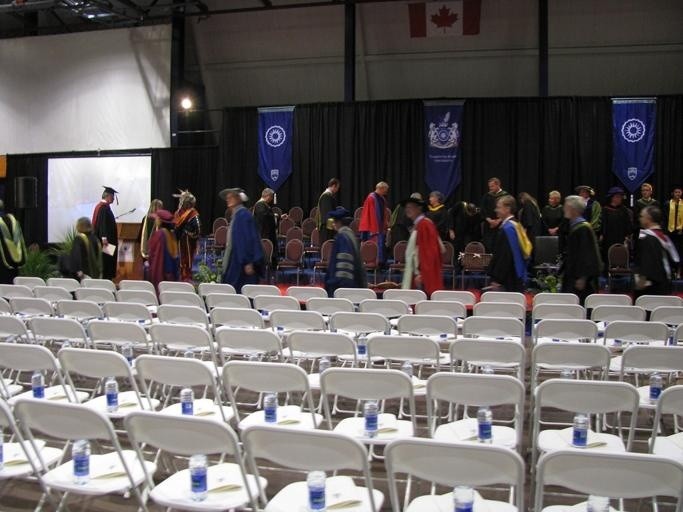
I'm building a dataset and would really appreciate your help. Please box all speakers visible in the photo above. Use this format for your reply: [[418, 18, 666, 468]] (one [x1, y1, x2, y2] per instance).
[[19, 175, 39, 208]]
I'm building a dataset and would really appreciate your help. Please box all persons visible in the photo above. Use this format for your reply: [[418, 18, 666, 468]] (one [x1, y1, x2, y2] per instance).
[[317, 177, 483, 298], [0, 198, 27, 284], [70, 176, 282, 297], [485, 176, 682, 299]]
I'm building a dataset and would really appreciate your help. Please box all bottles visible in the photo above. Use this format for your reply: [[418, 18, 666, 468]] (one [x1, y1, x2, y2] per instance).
[[187, 455, 210, 503], [104, 375, 122, 411], [180, 385, 194, 416], [475, 405, 493, 444], [261, 389, 279, 423], [1, 300, 676, 379], [363, 399, 379, 439], [648, 368, 664, 409], [585, 492, 612, 512], [451, 481, 474, 512], [305, 469, 328, 510], [30, 368, 47, 398], [573, 412, 587, 448], [69, 438, 90, 486]]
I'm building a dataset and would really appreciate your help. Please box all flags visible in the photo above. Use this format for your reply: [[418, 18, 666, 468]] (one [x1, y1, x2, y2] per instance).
[[408, 0, 481, 38]]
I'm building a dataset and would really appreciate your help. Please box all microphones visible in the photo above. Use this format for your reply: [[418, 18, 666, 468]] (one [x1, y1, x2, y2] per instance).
[[115, 207, 137, 220]]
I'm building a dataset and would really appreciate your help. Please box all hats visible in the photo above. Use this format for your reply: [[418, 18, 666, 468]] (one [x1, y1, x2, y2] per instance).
[[172, 190, 196, 204], [607, 187, 627, 194], [104, 185, 119, 205], [221, 187, 250, 202], [156, 210, 174, 222], [327, 209, 354, 221], [575, 185, 595, 195], [399, 192, 427, 210]]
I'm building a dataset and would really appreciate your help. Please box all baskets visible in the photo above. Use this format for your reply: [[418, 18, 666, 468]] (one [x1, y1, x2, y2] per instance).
[[461, 253, 492, 266]]
